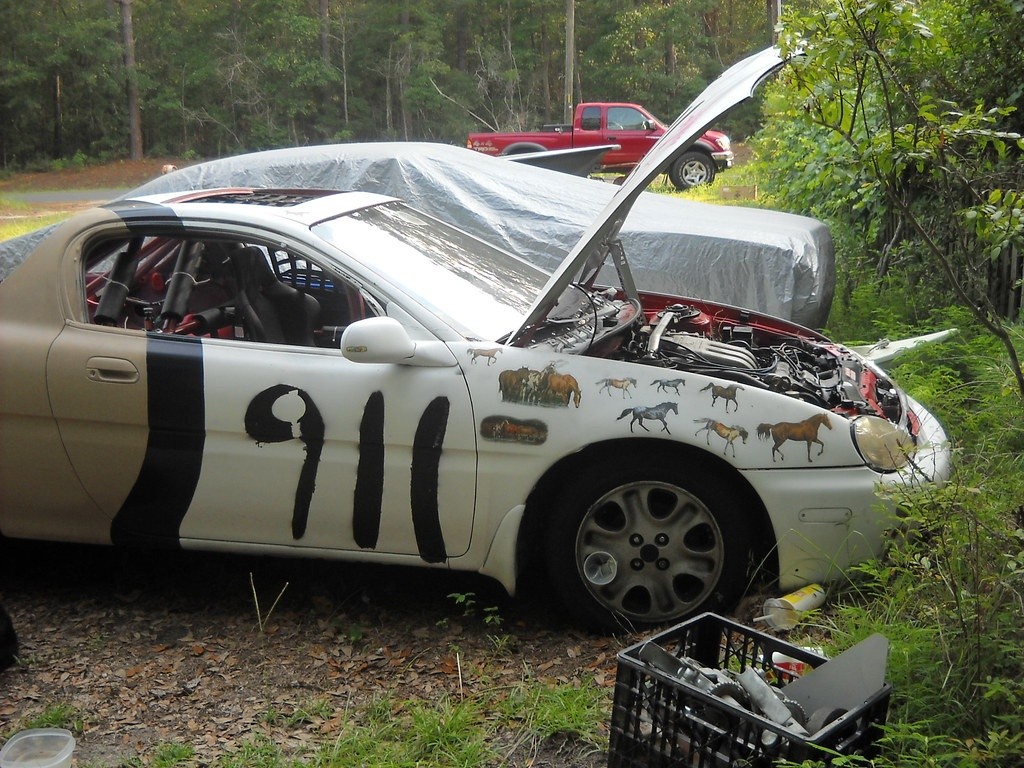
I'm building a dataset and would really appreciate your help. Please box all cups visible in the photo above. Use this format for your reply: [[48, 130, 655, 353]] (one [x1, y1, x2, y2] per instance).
[[763, 584, 826, 631]]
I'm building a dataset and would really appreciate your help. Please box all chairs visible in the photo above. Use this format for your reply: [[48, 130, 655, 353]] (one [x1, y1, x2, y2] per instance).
[[222, 246, 323, 346]]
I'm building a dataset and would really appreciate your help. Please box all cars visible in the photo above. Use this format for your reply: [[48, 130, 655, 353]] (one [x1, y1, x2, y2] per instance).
[[0, 46, 951, 639]]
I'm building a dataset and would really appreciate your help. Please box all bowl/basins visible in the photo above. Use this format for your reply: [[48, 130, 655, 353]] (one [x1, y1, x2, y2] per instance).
[[0, 728, 75, 768]]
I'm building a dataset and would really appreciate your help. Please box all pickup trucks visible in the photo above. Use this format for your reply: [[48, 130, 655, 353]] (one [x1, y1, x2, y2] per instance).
[[466, 101, 734, 191]]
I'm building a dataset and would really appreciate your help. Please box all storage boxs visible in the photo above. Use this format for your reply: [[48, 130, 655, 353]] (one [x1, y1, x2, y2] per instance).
[[607, 612, 892, 768]]
